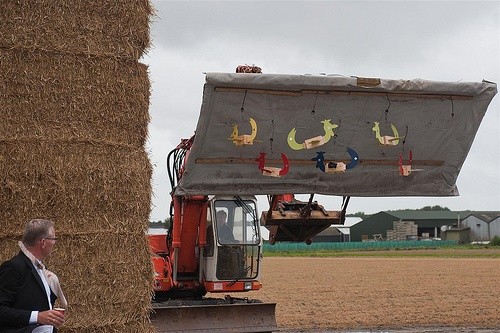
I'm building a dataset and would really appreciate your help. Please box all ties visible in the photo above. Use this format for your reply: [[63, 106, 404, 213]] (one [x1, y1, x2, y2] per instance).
[[18, 241, 68, 315]]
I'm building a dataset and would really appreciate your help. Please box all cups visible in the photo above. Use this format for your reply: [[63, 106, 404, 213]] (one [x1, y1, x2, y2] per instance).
[[54, 308, 65, 316]]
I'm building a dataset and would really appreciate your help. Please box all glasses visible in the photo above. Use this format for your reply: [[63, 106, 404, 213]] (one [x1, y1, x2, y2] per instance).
[[46, 237, 59, 244]]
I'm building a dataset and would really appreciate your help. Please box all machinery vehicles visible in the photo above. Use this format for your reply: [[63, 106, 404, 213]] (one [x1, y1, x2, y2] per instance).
[[146, 63, 500, 333]]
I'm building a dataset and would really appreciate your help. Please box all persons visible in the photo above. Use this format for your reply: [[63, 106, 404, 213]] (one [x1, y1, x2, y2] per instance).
[[0, 218, 68, 333], [206, 210, 244, 257]]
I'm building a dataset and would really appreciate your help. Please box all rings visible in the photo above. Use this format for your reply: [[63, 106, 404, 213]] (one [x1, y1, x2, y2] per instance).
[[53, 319, 56, 321]]
